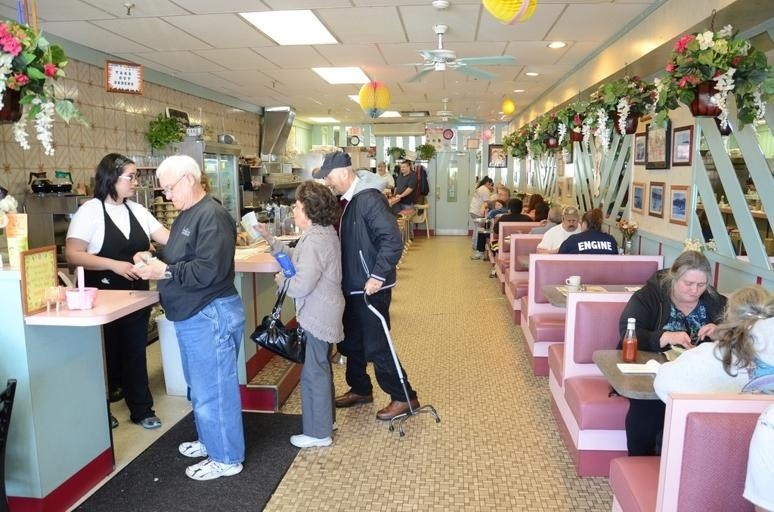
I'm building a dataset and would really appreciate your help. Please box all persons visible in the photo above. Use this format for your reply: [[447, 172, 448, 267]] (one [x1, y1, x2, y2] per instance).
[[697, 208, 713, 242], [62, 153, 174, 431], [312, 151, 421, 420], [608, 250, 727, 456], [130, 154, 243, 483], [393, 160, 418, 241], [374, 161, 395, 191], [744, 177, 757, 197], [199, 172, 223, 206], [253, 180, 346, 448], [467, 175, 619, 279], [651, 284, 774, 402]]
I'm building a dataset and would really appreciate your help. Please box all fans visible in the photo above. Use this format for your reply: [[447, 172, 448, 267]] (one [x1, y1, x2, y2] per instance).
[[391, 23, 524, 85], [409, 98, 490, 126]]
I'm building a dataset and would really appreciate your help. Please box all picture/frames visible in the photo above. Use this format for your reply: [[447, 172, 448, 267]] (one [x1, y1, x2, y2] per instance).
[[487, 144, 508, 168], [19, 243, 60, 316], [631, 116, 694, 227]]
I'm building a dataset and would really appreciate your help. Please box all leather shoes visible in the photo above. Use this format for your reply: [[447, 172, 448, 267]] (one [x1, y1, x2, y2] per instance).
[[334, 390, 374, 407], [376, 395, 421, 419]]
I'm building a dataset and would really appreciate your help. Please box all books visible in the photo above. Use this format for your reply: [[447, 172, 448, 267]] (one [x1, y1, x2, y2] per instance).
[[661, 343, 687, 360], [616, 359, 662, 374]]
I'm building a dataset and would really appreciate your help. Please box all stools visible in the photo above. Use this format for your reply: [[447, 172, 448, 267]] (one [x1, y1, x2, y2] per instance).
[[394, 206, 419, 271]]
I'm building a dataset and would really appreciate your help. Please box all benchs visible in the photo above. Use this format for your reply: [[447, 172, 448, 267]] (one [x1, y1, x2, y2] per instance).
[[485, 212, 774, 512]]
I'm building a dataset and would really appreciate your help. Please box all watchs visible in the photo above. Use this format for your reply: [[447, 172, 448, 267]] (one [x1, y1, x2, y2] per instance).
[[163, 264, 172, 280]]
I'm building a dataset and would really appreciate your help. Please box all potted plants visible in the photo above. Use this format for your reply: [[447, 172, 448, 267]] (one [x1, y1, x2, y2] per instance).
[[387, 145, 406, 162], [415, 144, 437, 160]]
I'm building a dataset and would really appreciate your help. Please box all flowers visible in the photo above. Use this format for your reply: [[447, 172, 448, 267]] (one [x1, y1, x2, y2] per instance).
[[501, 67, 658, 161], [654, 23, 772, 132], [0, 19, 92, 157]]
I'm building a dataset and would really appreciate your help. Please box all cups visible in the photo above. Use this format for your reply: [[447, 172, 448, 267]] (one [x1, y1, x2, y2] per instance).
[[66, 287, 97, 310], [566, 275, 581, 287], [274, 252, 296, 279]]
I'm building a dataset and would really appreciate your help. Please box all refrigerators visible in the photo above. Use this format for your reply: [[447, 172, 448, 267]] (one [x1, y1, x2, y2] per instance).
[[150, 140, 239, 235]]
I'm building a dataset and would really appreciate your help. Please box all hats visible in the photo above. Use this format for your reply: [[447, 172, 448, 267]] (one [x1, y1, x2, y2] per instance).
[[563, 207, 580, 220], [315, 151, 351, 179]]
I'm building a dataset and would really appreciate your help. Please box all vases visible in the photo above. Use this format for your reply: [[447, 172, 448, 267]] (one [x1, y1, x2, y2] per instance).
[[0, 86, 22, 124], [688, 80, 726, 119]]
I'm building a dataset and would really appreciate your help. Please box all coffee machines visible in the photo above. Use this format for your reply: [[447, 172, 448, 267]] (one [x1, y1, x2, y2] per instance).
[[24, 194, 93, 266]]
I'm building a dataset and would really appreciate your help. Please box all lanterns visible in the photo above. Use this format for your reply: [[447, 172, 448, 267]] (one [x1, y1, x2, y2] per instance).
[[480, 0, 537, 26], [358, 81, 389, 122]]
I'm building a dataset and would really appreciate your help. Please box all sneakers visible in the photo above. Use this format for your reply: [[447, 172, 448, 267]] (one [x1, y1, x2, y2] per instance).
[[490, 267, 497, 278], [185, 456, 244, 482], [470, 253, 484, 259], [179, 439, 207, 458], [291, 433, 333, 448], [140, 415, 162, 428]]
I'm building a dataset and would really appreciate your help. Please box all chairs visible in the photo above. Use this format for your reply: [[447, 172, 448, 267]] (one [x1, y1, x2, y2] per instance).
[[411, 201, 430, 239], [0, 377, 17, 512]]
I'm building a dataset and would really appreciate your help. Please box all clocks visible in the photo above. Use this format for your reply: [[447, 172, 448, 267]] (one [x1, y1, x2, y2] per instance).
[[350, 136, 360, 146], [442, 129, 454, 140], [218, 134, 236, 144]]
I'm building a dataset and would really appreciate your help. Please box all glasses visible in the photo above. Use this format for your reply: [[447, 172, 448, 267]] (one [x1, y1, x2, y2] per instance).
[[162, 175, 185, 195], [119, 169, 141, 182]]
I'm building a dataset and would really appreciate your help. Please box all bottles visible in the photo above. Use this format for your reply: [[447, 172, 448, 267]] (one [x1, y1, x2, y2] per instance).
[[623, 318, 638, 364]]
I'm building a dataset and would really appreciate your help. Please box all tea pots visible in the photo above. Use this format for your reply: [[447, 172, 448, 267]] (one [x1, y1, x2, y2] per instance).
[[51, 171, 73, 193], [26, 170, 51, 194]]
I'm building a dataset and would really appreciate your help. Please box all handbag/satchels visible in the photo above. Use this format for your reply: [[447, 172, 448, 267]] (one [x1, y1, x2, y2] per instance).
[[249, 279, 305, 364]]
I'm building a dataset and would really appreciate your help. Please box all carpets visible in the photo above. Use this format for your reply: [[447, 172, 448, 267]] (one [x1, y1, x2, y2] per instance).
[[71, 409, 303, 512]]
